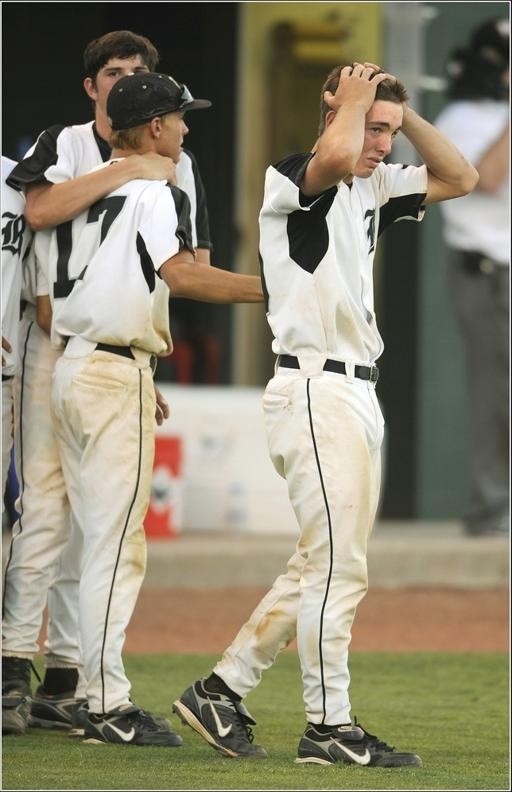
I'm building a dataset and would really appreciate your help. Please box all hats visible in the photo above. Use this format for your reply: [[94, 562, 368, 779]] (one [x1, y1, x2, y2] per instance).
[[468, 14, 508, 69], [107, 72, 212, 128]]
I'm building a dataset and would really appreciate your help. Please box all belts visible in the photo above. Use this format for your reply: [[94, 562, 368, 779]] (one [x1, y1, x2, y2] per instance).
[[277, 353, 381, 383], [459, 251, 509, 280], [66, 335, 135, 358]]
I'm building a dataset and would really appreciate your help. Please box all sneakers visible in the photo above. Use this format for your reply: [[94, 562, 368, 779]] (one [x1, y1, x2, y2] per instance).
[[28, 685, 90, 730], [293, 722, 423, 769], [68, 704, 184, 746], [3, 676, 29, 734], [172, 677, 267, 759]]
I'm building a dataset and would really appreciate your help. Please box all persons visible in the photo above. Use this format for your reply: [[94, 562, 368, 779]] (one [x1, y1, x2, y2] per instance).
[[35, 72, 264, 748], [1, 155, 34, 515], [173, 61, 482, 768], [434, 16, 510, 538], [2, 31, 213, 735]]
